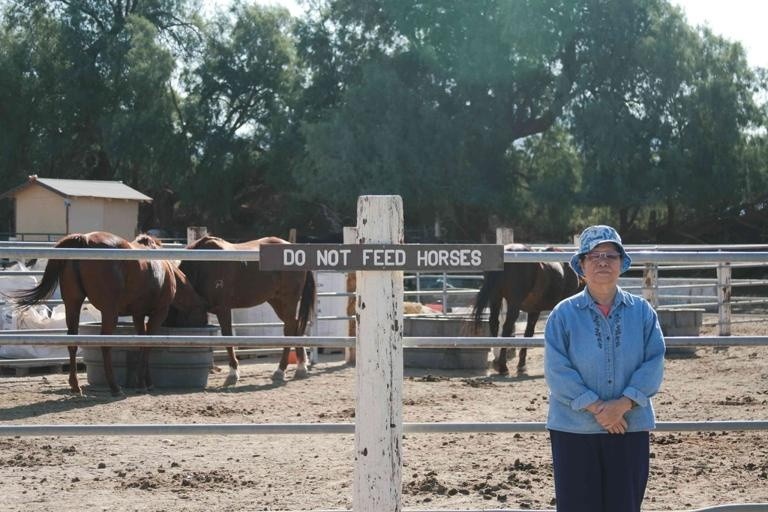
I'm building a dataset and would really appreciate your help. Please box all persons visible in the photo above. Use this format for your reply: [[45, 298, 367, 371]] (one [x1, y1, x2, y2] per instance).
[[543, 225, 667, 512]]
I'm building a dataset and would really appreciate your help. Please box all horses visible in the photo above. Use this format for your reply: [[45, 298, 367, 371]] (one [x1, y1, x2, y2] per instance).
[[471, 248, 587, 376], [163, 235, 318, 386], [4, 231, 207, 401]]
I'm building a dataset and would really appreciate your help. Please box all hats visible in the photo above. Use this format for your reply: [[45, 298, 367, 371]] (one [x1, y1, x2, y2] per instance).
[[568, 224, 633, 280]]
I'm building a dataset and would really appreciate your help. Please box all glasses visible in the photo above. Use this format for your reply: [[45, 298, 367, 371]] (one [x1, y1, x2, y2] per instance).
[[583, 250, 624, 261]]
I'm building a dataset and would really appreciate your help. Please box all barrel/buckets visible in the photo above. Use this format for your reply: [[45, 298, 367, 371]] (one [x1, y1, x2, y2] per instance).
[[145, 324, 222, 389], [653, 309, 706, 355], [77, 321, 147, 386]]
